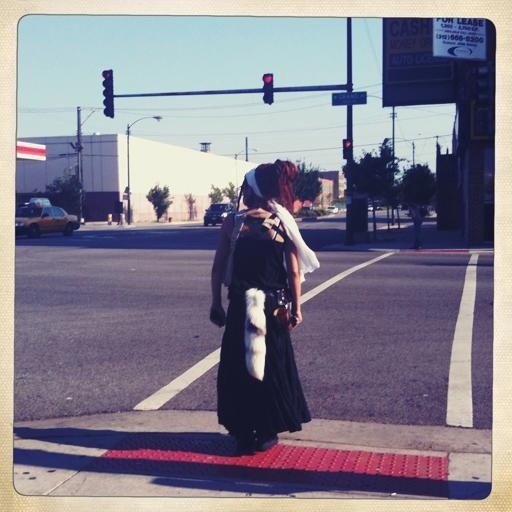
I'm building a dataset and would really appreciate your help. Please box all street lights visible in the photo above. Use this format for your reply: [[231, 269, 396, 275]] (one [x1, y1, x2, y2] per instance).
[[126, 115, 163, 225], [235, 148, 259, 190]]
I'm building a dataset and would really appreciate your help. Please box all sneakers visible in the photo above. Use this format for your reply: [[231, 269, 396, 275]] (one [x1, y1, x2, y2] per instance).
[[233, 432, 279, 451]]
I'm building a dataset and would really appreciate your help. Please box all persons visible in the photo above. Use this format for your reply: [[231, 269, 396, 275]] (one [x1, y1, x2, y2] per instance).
[[209, 160, 320, 451], [407, 204, 426, 249], [115, 201, 123, 226]]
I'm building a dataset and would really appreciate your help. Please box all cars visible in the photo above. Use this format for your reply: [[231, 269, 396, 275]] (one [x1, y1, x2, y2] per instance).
[[327, 206, 338, 214], [15, 198, 80, 238], [204, 204, 237, 226]]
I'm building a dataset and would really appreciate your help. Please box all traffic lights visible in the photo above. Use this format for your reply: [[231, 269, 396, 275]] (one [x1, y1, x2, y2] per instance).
[[102, 69, 114, 118], [342, 139, 352, 159], [263, 74, 274, 103]]
